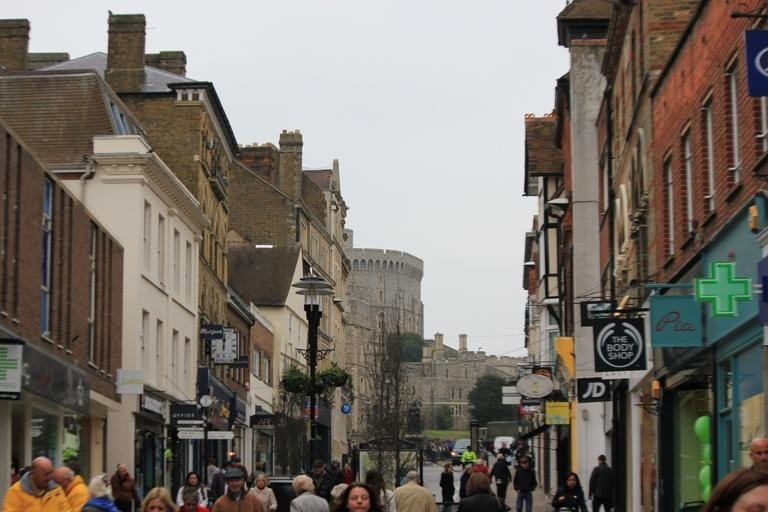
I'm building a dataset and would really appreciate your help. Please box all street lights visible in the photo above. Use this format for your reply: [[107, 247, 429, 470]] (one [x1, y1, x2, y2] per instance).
[[290, 266, 334, 490], [365, 394, 374, 435]]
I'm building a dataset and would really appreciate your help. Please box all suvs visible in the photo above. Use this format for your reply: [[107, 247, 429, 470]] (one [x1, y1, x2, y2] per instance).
[[450, 438, 482, 465]]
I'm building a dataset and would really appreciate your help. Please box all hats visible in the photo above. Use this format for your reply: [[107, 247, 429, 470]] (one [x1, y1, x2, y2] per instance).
[[89, 474, 113, 497], [222, 469, 246, 480]]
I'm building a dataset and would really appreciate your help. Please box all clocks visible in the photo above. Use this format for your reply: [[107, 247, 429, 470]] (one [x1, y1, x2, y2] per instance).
[[205, 325, 240, 367]]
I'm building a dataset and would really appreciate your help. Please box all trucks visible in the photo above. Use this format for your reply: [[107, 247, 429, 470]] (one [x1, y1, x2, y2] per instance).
[[477, 420, 519, 445], [494, 435, 516, 457]]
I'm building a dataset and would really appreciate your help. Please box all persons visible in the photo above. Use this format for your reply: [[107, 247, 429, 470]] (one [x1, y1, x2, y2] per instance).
[[3, 443, 538, 512], [700, 469, 768, 512], [749, 437, 768, 464], [552, 472, 587, 512], [589, 454, 614, 512]]
